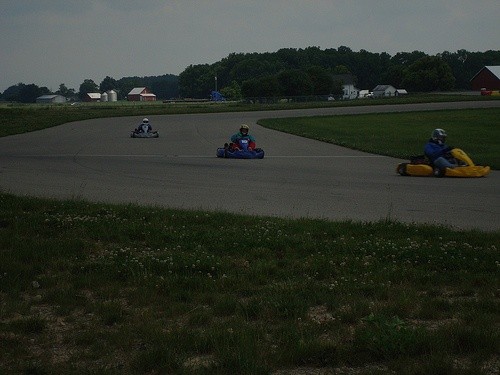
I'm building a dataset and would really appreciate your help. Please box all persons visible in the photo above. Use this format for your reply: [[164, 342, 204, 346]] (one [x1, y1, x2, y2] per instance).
[[138, 118, 152, 133], [424, 129, 467, 167], [231, 124, 256, 151]]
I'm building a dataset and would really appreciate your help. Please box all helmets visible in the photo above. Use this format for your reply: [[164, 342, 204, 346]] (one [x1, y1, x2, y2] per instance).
[[431, 129, 447, 145], [240, 124, 249, 136], [142, 118, 149, 125]]
